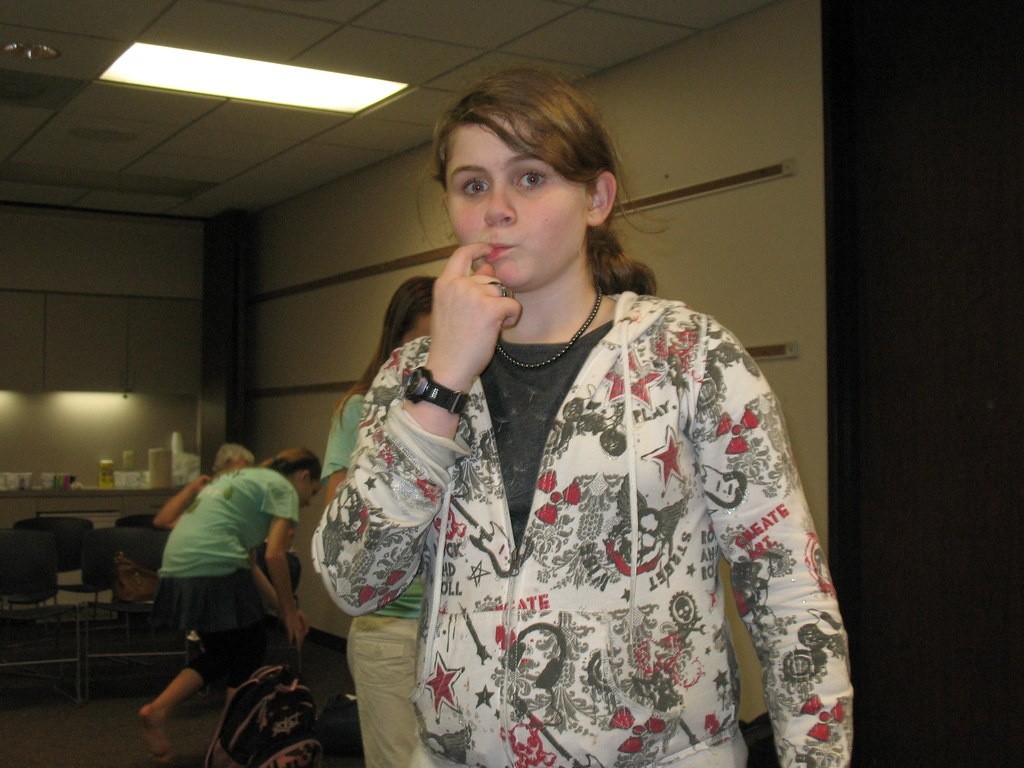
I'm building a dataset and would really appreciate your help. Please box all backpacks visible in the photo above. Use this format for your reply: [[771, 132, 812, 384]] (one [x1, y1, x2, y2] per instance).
[[205, 666, 323, 768]]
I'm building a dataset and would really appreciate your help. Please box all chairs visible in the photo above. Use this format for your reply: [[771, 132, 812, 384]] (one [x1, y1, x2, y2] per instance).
[[0, 515, 302, 707]]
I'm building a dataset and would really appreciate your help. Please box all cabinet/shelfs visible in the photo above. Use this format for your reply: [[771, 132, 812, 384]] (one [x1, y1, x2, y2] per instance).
[[0, 495, 171, 529]]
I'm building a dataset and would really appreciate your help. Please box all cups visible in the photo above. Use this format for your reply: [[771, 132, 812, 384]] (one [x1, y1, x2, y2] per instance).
[[1, 473, 18, 490], [147, 449, 173, 489], [99, 460, 114, 489], [20, 472, 33, 490]]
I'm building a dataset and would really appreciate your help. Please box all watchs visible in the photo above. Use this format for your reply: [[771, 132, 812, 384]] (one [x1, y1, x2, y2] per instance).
[[405, 365, 474, 417]]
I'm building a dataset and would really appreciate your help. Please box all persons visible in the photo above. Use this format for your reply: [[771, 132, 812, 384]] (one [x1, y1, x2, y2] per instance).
[[317, 276, 436, 758], [140, 440, 322, 765], [312, 67, 854, 766]]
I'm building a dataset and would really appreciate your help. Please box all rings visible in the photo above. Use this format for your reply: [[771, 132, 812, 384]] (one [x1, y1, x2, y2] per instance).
[[500, 285, 514, 297]]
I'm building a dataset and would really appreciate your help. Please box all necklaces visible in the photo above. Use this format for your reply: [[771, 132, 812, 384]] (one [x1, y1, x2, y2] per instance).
[[494, 280, 603, 368]]
